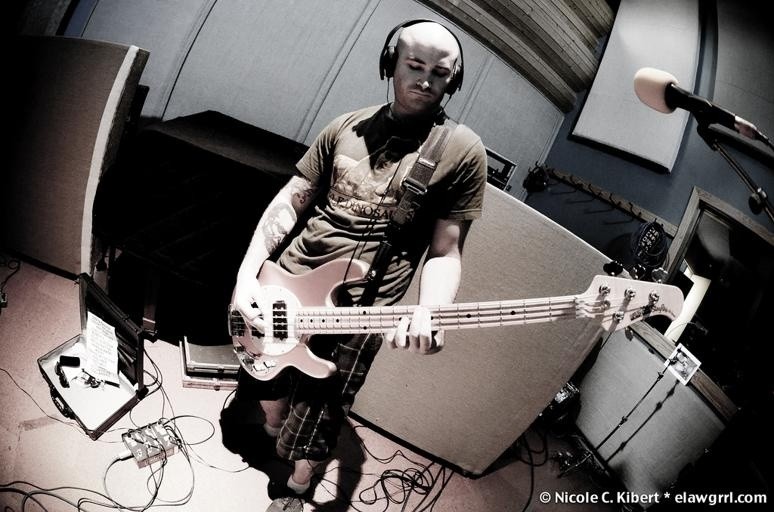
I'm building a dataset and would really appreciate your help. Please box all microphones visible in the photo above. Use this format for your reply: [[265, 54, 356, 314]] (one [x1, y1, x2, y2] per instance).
[[632, 68, 770, 147]]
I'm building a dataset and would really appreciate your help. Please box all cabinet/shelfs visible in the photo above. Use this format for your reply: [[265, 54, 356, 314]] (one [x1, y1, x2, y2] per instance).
[[570, 316, 730, 511]]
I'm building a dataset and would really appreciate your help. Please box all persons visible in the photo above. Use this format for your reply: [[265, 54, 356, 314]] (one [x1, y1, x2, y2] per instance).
[[228, 19, 488, 502]]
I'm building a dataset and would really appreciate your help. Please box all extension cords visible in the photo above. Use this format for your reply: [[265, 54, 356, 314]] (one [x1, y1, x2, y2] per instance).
[[121, 419, 177, 469]]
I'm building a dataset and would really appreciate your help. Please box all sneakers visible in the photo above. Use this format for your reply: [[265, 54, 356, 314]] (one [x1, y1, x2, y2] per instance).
[[265, 485, 303, 512]]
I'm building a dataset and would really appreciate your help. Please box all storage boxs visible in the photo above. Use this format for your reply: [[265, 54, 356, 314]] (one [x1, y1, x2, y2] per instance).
[[182, 335, 240, 376], [36, 272, 149, 441], [177, 340, 240, 391]]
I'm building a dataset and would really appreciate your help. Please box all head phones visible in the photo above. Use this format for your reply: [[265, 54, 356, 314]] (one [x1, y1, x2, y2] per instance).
[[379, 20, 465, 98]]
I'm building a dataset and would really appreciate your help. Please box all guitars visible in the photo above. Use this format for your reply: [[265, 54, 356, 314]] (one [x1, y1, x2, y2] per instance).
[[226, 257, 683, 382]]
